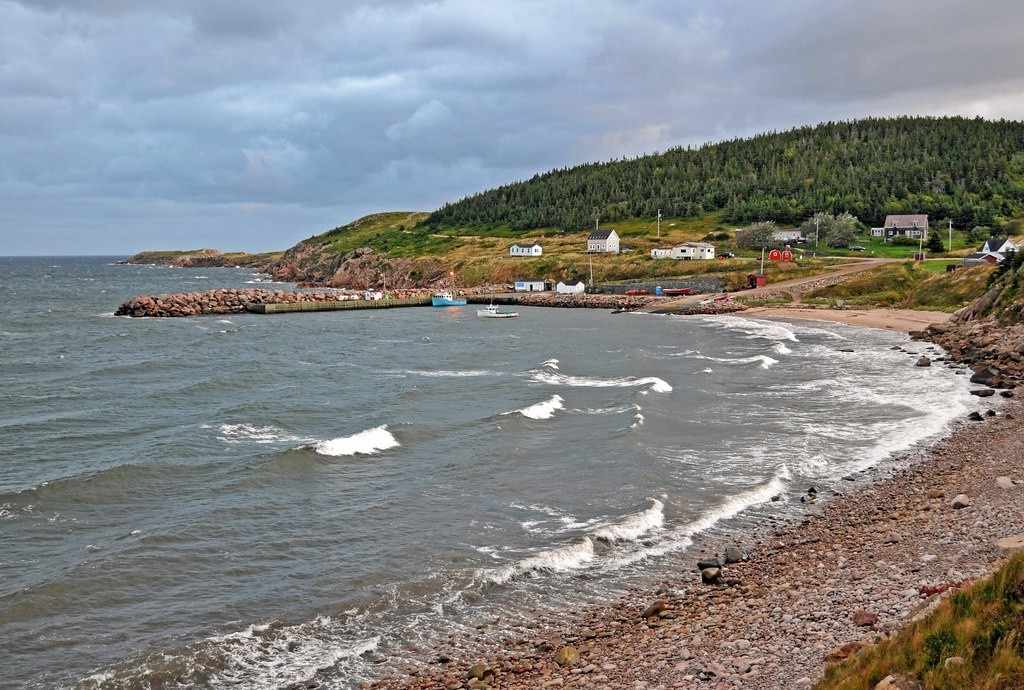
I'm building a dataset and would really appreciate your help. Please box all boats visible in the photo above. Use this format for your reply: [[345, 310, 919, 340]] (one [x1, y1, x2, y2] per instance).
[[431, 289, 466, 306], [477, 303, 518, 318]]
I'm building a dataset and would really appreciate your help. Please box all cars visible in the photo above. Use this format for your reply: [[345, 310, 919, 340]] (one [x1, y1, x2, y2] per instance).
[[848, 245, 865, 251], [717, 253, 731, 258]]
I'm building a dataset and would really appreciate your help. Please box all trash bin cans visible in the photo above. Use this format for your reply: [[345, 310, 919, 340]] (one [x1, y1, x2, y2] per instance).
[[655, 286, 662, 295]]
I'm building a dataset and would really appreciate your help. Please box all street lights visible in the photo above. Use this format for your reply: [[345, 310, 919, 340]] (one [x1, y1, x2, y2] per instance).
[[657, 209, 661, 238], [381, 273, 385, 292], [450, 269, 455, 295], [760, 247, 766, 275]]
